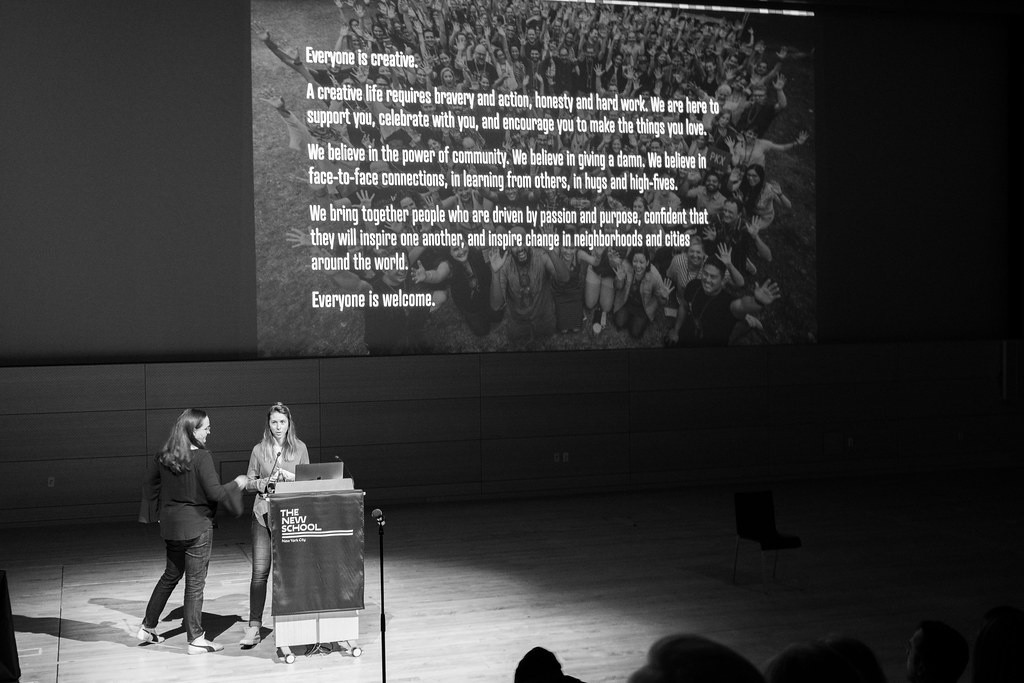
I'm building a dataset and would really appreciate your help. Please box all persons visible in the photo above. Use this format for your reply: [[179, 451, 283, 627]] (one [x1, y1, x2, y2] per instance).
[[249, 0, 809, 346], [136, 407, 250, 657], [240, 401, 312, 647], [515, 604, 1024, 683]]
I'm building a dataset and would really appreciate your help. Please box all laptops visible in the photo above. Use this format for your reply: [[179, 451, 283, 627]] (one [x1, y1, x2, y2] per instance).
[[295, 462, 344, 481]]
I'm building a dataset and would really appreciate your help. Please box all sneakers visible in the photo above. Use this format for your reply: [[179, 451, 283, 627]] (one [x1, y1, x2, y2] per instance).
[[240, 628, 260, 647], [188, 631, 225, 655], [138, 628, 164, 644]]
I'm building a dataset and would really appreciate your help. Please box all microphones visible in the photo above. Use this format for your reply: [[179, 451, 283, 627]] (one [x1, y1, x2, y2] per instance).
[[266, 452, 281, 493], [335, 456, 352, 478], [371, 509, 386, 526]]
